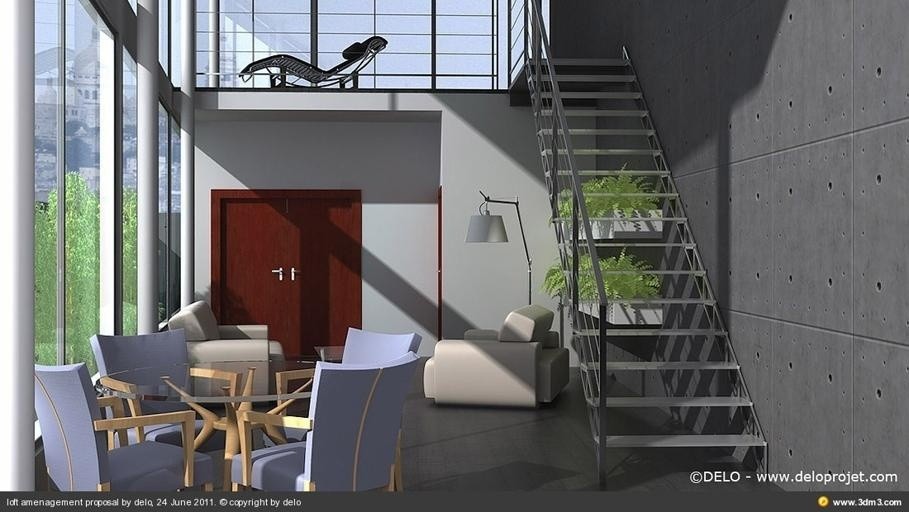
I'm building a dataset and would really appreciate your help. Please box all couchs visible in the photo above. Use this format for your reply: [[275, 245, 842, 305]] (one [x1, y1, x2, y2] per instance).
[[166, 300, 286, 406], [422, 303, 571, 408]]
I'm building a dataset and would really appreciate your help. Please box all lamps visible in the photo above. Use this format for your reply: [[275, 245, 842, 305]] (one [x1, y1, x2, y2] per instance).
[[464, 191, 532, 308]]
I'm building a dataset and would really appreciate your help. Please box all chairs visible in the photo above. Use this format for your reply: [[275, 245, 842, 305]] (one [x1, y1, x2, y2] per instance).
[[89, 327, 246, 452], [239, 36, 389, 92], [277, 326, 422, 416], [230, 350, 421, 493], [34, 360, 215, 498]]
